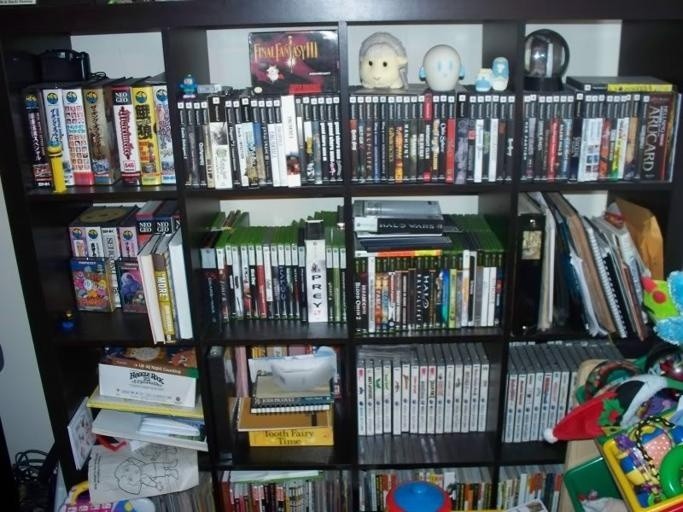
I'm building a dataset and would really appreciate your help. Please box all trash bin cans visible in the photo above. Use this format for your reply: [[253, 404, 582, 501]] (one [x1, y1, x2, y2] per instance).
[[10, 465, 56, 511], [386, 480, 452, 511]]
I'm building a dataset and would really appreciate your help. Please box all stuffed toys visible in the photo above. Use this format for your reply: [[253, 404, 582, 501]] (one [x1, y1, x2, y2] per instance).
[[642, 270, 682, 347]]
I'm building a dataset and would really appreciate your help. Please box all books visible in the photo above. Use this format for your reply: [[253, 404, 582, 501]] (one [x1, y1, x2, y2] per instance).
[[199, 203, 347, 324], [234, 344, 342, 447], [220, 470, 353, 512], [497, 465, 565, 512], [521, 75, 681, 183], [348, 82, 516, 184], [352, 199, 504, 334], [24, 71, 177, 191], [506, 341, 625, 443], [69, 200, 194, 346], [88, 387, 209, 453], [147, 472, 216, 512], [357, 467, 492, 512], [177, 86, 343, 190], [355, 344, 490, 435], [517, 190, 653, 340]]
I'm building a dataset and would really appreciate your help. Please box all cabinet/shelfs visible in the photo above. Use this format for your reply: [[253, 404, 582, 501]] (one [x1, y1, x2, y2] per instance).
[[0, 1, 682, 512]]
